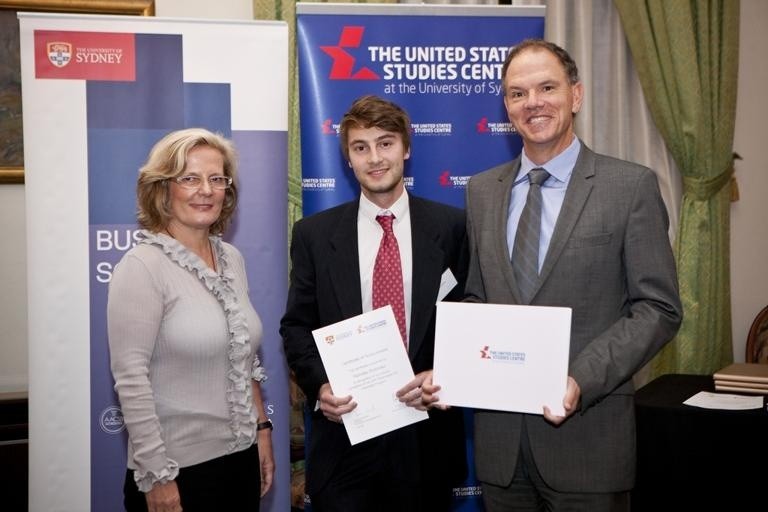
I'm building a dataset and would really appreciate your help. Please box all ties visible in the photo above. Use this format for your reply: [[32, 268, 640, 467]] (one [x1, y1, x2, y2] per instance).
[[371, 214, 408, 352], [510, 167, 551, 305]]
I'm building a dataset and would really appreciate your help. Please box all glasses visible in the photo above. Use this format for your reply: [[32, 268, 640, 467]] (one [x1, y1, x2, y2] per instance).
[[168, 176, 233, 190]]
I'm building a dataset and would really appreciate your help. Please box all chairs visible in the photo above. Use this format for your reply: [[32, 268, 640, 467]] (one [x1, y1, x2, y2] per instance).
[[745, 305, 768, 364]]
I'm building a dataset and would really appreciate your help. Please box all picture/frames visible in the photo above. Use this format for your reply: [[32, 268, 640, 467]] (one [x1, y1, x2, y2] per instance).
[[0, 0, 155, 183]]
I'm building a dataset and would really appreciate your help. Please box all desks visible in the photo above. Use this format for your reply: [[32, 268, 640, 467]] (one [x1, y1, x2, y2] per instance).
[[633, 374, 768, 512]]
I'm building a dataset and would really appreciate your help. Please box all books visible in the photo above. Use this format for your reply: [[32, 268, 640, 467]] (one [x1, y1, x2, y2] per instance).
[[712, 362, 768, 396]]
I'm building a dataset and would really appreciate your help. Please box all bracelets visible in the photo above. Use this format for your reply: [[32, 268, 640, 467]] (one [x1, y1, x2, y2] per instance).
[[255, 418, 274, 433]]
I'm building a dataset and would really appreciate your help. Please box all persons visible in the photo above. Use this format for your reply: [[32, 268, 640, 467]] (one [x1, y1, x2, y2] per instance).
[[461, 37, 685, 511], [275, 92, 470, 512], [104, 125, 277, 512]]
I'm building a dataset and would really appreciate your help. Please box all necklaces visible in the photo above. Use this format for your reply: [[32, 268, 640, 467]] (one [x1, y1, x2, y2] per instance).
[[163, 226, 216, 273]]
